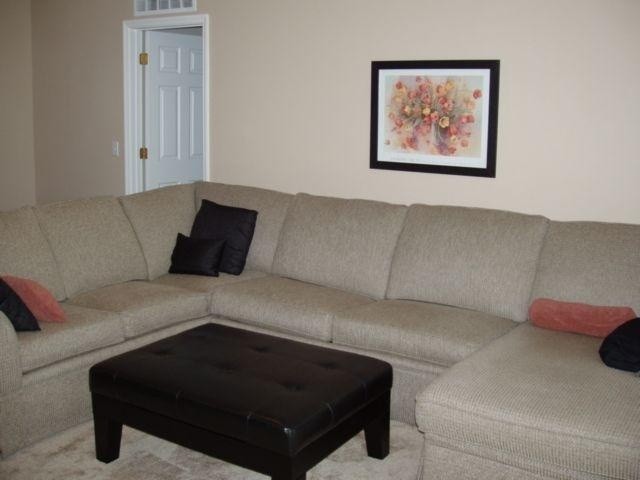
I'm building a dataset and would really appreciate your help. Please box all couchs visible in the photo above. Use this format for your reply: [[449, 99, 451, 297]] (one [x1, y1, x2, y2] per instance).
[[0, 180, 639, 480]]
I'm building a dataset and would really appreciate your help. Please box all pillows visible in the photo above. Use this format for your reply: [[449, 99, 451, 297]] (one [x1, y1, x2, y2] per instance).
[[167, 198, 258, 278], [530, 293, 639, 372], [0, 274, 67, 332]]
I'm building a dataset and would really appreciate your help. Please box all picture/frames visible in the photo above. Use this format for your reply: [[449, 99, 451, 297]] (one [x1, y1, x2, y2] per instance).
[[368, 59, 501, 179]]
